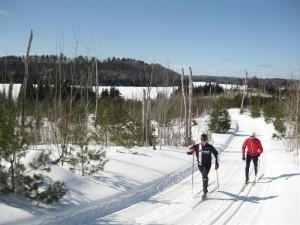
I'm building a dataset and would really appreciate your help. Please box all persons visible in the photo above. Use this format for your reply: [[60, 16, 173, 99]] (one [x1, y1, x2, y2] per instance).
[[242, 132, 264, 184], [186, 133, 220, 200]]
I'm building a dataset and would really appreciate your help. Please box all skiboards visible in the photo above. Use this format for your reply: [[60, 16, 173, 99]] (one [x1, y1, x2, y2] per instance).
[[241, 164, 260, 192], [191, 179, 220, 209]]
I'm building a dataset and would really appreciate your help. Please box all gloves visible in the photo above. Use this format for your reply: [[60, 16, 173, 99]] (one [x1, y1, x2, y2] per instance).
[[256, 153, 260, 157], [242, 156, 245, 160]]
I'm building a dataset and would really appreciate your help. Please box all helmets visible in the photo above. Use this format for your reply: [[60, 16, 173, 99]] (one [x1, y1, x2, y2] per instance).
[[201, 134, 207, 139]]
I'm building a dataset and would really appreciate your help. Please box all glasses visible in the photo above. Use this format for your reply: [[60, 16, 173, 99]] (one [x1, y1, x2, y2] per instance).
[[202, 140, 206, 142]]
